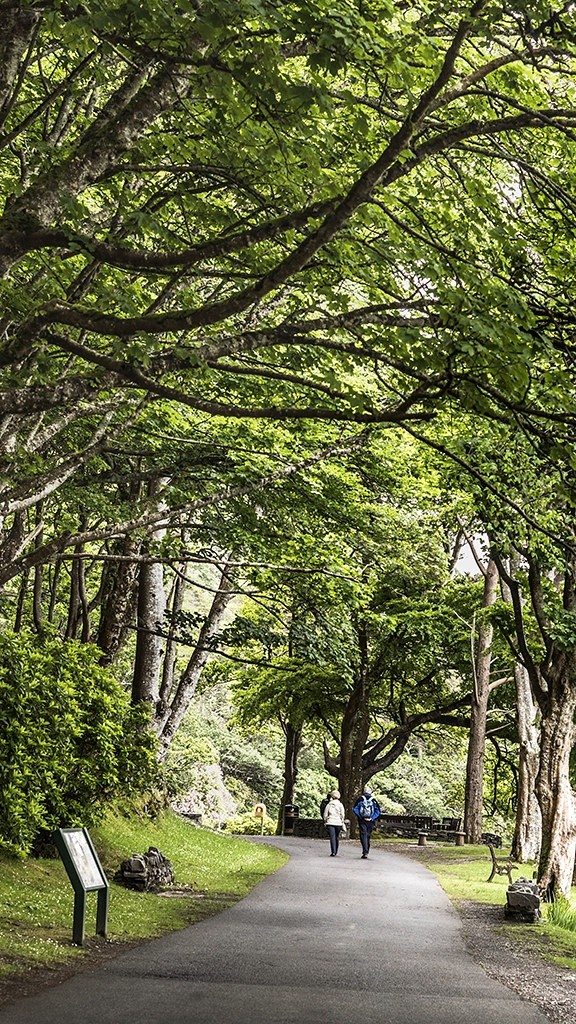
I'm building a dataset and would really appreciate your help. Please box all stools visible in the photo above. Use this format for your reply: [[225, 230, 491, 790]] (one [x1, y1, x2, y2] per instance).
[[417, 832, 428, 846], [455, 831, 466, 845]]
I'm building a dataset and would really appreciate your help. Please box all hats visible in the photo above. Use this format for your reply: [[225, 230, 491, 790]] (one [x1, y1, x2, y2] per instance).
[[328, 790, 340, 799], [363, 787, 372, 794]]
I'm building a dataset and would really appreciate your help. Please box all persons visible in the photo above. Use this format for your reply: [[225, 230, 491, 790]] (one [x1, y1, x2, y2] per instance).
[[319, 790, 345, 857], [353, 786, 381, 858]]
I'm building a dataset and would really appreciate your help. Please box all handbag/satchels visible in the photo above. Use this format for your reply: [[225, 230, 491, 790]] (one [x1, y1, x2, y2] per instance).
[[342, 823, 347, 832]]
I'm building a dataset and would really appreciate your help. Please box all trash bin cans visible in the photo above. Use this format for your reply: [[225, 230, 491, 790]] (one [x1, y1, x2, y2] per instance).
[[282, 804, 300, 836]]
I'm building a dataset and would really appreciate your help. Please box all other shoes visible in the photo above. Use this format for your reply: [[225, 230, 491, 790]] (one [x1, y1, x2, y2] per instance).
[[361, 854, 367, 859], [330, 853, 336, 856]]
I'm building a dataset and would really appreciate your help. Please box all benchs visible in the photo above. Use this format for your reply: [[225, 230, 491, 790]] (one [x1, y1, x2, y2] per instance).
[[433, 817, 462, 840], [486, 843, 519, 884]]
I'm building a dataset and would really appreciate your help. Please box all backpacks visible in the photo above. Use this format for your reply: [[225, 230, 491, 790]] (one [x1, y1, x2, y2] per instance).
[[360, 795, 374, 819]]
